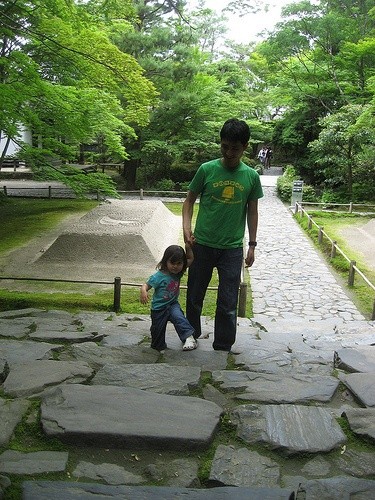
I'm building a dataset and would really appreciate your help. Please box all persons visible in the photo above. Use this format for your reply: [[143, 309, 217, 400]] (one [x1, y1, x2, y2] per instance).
[[182, 117, 264, 351], [141, 236, 200, 351], [257, 145, 273, 169]]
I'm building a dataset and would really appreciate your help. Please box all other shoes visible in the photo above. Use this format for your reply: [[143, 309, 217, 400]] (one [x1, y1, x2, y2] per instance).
[[182, 339, 198, 350]]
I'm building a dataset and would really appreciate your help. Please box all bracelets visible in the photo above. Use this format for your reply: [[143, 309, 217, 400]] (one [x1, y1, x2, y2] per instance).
[[249, 241, 257, 246]]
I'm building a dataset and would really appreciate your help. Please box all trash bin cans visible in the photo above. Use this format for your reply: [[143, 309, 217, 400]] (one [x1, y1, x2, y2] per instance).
[[288, 178, 304, 209]]
